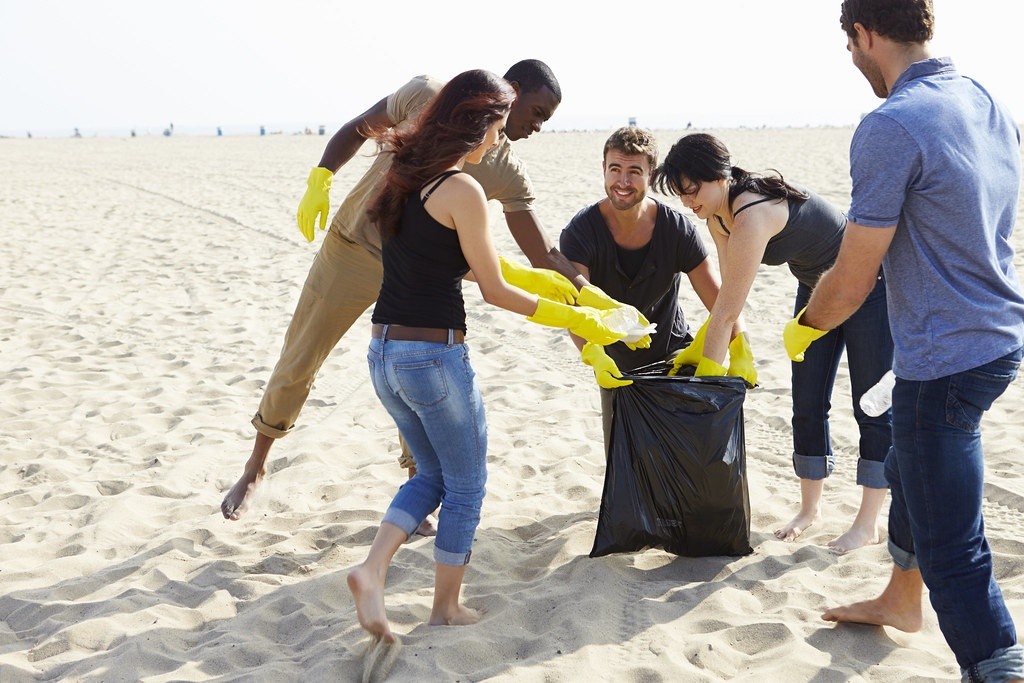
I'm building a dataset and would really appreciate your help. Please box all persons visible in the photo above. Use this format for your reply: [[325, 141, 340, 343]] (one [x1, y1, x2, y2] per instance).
[[649, 134, 900, 554], [218, 57, 654, 522], [342, 68, 656, 644], [558, 125, 755, 488], [779, 0, 1024, 683]]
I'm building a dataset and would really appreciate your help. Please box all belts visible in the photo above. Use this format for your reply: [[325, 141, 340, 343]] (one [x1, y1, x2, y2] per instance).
[[372, 324, 464, 344]]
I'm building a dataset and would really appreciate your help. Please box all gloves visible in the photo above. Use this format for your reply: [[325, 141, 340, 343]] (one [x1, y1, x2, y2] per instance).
[[576, 284, 652, 351], [783, 304, 829, 361], [297, 166, 334, 242], [581, 341, 633, 388], [667, 315, 728, 377], [727, 331, 758, 386], [526, 298, 628, 345], [499, 256, 579, 305]]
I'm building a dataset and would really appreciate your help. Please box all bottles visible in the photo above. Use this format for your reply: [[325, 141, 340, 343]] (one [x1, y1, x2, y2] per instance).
[[859, 370, 897, 418], [603, 307, 639, 333]]
